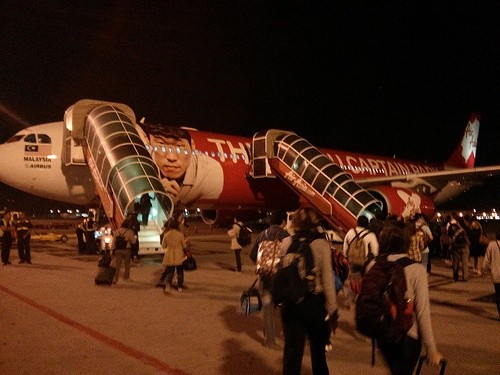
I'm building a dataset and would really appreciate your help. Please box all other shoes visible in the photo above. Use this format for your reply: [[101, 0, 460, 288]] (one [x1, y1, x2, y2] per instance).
[[165, 282, 171, 292], [178, 287, 183, 292], [18, 260, 32, 264]]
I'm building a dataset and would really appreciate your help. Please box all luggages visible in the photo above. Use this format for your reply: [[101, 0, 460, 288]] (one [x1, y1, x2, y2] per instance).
[[95, 266, 116, 286], [97, 253, 112, 267]]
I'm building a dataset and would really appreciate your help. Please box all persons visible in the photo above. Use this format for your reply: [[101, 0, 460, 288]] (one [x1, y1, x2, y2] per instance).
[[227, 214, 246, 273], [137, 120, 224, 207], [127, 199, 138, 217], [76, 213, 99, 255], [140, 193, 152, 226], [13, 212, 33, 264], [277, 208, 339, 375], [156, 211, 191, 294], [250, 210, 292, 352], [411, 211, 500, 316], [363, 223, 443, 374], [0, 211, 13, 266], [109, 213, 141, 283], [341, 215, 379, 299]]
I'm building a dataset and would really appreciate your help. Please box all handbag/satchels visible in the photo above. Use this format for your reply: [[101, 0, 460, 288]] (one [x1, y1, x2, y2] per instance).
[[183, 256, 197, 271], [240, 290, 262, 316]]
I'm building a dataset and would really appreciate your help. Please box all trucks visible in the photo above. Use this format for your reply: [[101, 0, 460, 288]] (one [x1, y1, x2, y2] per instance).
[[0, 210, 84, 242]]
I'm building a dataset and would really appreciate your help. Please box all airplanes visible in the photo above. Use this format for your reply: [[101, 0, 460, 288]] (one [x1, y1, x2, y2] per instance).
[[0, 78, 500, 257]]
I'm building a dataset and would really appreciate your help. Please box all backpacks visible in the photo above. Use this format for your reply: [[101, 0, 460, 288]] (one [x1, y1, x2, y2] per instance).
[[116, 228, 129, 249], [416, 224, 427, 251], [355, 253, 421, 339], [330, 247, 349, 294], [269, 232, 325, 308], [256, 227, 285, 278], [346, 228, 371, 267], [379, 225, 403, 254], [233, 223, 252, 247]]
